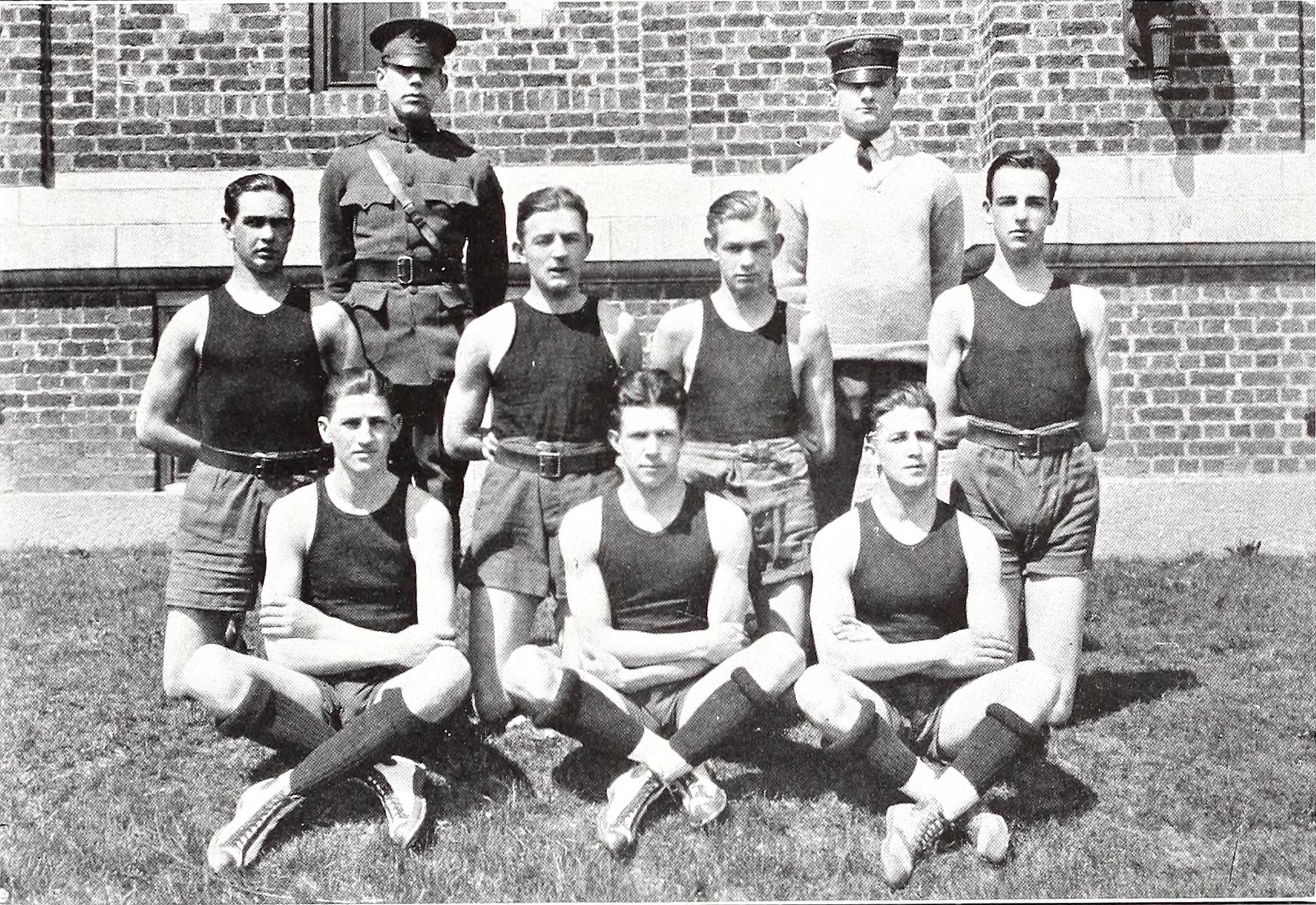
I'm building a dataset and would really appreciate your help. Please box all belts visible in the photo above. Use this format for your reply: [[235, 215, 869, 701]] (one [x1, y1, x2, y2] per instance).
[[354, 256, 466, 287], [680, 437, 781, 459], [967, 420, 1085, 458], [197, 444, 325, 480], [494, 445, 616, 478]]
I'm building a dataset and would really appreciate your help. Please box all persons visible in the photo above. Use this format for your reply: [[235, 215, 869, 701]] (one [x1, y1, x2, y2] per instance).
[[502, 368, 806, 856], [316, 15, 509, 589], [647, 189, 836, 714], [925, 150, 1113, 723], [769, 34, 965, 528], [443, 188, 645, 723], [794, 379, 1063, 886], [136, 173, 370, 699], [179, 369, 475, 876]]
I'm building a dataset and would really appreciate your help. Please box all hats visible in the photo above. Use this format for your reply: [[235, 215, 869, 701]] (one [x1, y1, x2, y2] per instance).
[[824, 34, 904, 83], [369, 17, 457, 69]]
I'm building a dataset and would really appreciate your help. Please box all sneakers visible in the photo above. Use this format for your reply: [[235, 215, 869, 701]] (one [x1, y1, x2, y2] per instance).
[[959, 803, 1011, 864], [367, 754, 435, 848], [671, 763, 728, 828], [207, 778, 296, 876], [881, 799, 945, 889], [597, 763, 663, 858]]
